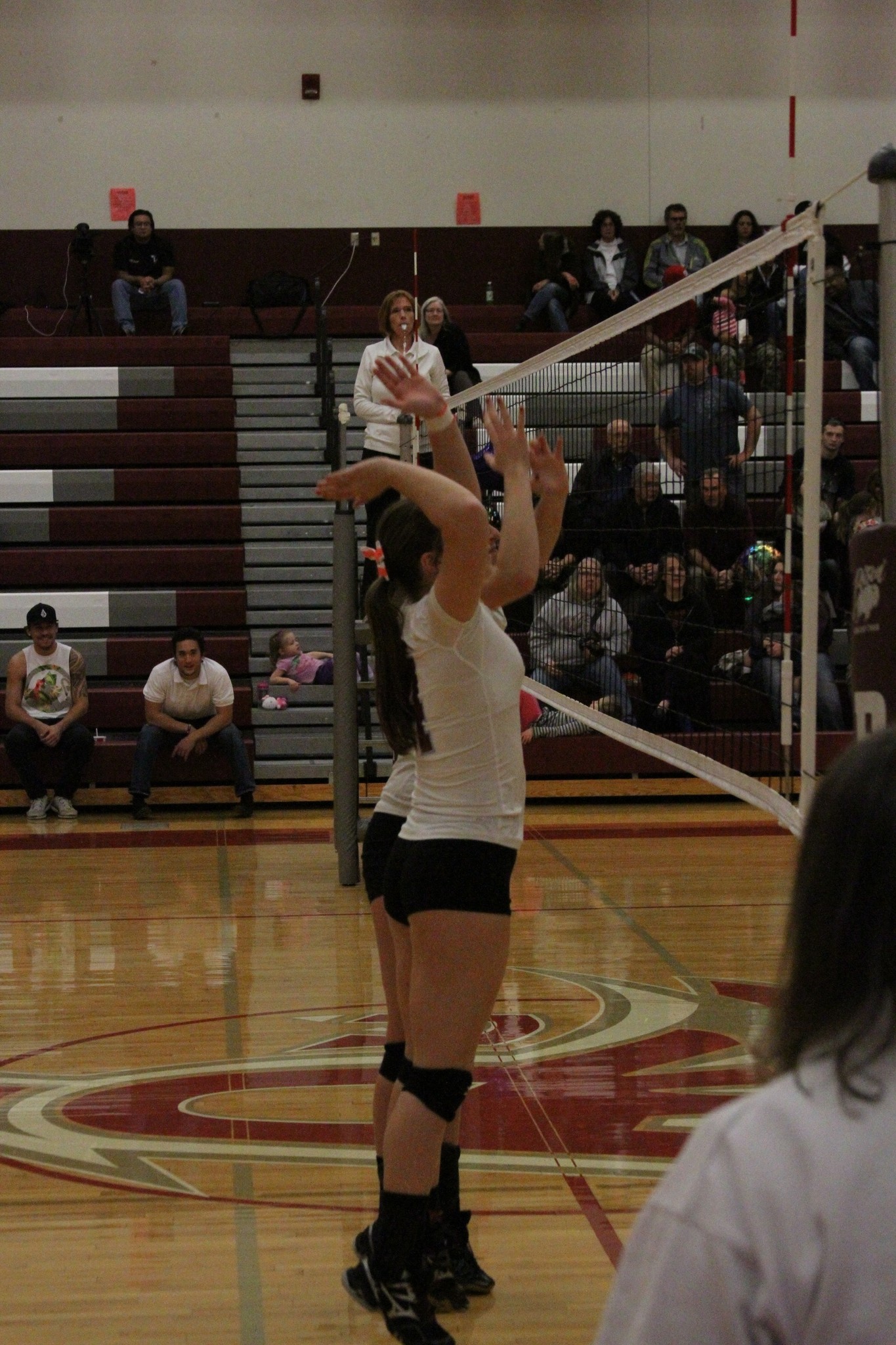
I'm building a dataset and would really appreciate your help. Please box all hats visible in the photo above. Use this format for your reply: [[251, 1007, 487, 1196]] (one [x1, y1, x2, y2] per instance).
[[679, 341, 708, 360], [26, 603, 59, 627]]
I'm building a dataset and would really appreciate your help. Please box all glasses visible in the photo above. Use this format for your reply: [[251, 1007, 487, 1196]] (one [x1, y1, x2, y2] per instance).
[[668, 216, 687, 221]]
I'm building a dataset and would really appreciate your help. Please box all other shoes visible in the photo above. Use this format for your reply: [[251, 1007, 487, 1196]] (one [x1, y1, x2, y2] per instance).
[[117, 326, 136, 336], [131, 803, 156, 820], [228, 803, 254, 817], [514, 319, 526, 331], [171, 324, 189, 336]]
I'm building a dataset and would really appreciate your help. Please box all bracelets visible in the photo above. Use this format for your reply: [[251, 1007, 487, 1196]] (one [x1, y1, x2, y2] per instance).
[[423, 405, 454, 435], [187, 724, 191, 735]]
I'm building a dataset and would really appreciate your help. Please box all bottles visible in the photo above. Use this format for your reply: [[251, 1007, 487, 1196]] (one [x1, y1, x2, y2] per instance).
[[256, 680, 270, 709], [485, 281, 494, 305]]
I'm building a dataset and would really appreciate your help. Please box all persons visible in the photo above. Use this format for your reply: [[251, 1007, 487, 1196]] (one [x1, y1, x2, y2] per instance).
[[352, 290, 452, 624], [111, 209, 189, 336], [317, 395, 541, 1345], [4, 603, 95, 820], [414, 295, 486, 428], [126, 627, 256, 819], [268, 631, 376, 693], [349, 351, 570, 1313], [579, 729, 896, 1345], [486, 200, 880, 746]]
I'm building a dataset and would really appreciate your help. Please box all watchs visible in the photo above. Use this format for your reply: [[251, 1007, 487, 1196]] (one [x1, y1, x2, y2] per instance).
[[401, 414, 412, 424]]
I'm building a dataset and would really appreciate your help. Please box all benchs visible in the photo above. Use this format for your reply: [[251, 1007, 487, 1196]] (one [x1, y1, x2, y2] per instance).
[[0, 306, 881, 803]]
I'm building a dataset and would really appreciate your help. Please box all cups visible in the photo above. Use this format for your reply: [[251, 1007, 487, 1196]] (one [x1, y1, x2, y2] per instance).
[[93, 735, 107, 742]]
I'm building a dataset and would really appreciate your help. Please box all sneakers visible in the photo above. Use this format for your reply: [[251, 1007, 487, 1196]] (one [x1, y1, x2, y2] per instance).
[[51, 796, 78, 818], [425, 1209, 495, 1314], [348, 1262, 458, 1345], [352, 1219, 440, 1314], [26, 794, 52, 820]]
